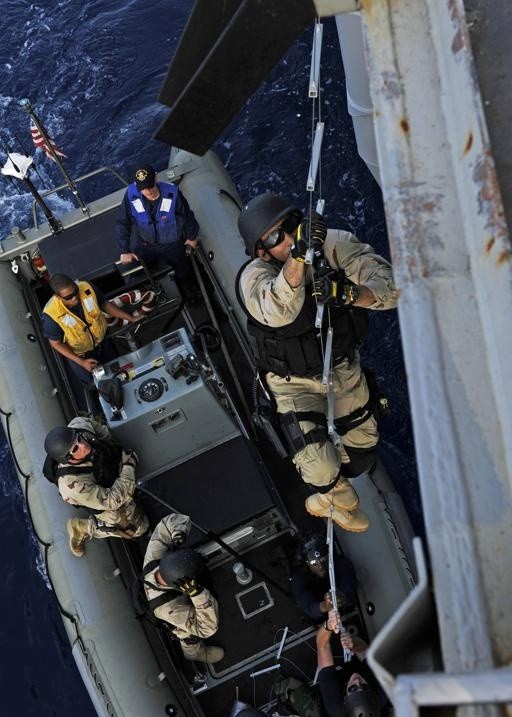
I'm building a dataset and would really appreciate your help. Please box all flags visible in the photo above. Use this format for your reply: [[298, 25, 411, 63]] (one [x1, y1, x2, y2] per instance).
[[30, 115, 69, 166]]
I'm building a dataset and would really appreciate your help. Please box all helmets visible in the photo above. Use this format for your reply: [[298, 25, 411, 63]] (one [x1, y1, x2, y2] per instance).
[[345, 693, 380, 717], [238, 192, 292, 259], [159, 549, 206, 588], [45, 426, 75, 464], [302, 538, 328, 561]]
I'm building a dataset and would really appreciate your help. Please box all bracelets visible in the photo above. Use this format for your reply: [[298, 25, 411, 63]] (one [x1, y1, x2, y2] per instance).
[[323, 618, 334, 631]]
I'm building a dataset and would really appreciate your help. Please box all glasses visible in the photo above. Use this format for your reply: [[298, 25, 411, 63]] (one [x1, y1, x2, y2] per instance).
[[348, 684, 369, 692], [262, 214, 300, 249], [60, 282, 79, 301], [69, 434, 81, 455], [305, 556, 328, 568]]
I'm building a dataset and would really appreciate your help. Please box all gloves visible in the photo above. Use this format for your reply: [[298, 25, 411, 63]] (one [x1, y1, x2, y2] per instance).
[[173, 576, 204, 596], [292, 214, 327, 263], [122, 448, 139, 467], [312, 267, 349, 306], [171, 530, 187, 549]]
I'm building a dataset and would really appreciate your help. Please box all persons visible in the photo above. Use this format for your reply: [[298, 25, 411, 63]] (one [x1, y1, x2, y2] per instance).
[[268, 607, 390, 717], [291, 533, 357, 619], [39, 272, 152, 404], [139, 512, 226, 664], [41, 415, 154, 560], [114, 163, 204, 310], [234, 192, 400, 533]]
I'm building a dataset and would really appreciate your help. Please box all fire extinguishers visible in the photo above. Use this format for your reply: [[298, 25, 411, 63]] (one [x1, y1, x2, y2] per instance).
[[31, 248, 49, 282]]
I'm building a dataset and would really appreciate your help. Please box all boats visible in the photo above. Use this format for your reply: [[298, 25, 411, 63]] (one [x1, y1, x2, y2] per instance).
[[1, 136, 458, 717]]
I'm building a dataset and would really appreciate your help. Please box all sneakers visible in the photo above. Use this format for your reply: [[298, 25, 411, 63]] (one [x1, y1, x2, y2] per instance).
[[326, 476, 361, 511], [304, 493, 370, 533]]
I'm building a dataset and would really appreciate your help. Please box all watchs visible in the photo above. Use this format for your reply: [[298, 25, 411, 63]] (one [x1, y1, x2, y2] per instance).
[[344, 278, 360, 307]]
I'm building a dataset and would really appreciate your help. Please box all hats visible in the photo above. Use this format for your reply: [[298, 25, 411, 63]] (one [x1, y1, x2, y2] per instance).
[[135, 165, 155, 192]]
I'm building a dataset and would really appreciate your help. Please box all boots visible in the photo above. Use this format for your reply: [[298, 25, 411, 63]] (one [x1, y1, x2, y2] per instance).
[[67, 518, 90, 557], [180, 639, 224, 664]]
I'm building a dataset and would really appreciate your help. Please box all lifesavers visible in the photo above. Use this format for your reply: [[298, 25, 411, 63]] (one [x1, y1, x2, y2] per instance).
[[102, 289, 158, 327]]
